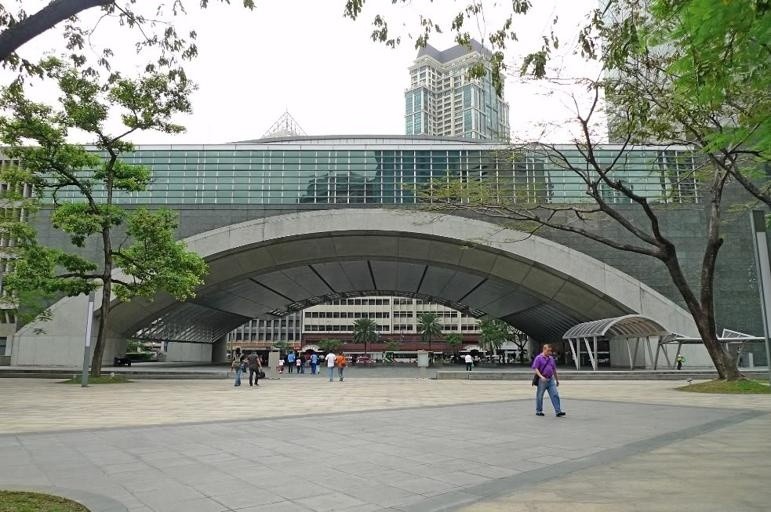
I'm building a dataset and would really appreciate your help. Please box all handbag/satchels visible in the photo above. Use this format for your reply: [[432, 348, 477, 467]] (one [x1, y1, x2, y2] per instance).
[[532, 375, 539, 386], [257, 372, 265, 379]]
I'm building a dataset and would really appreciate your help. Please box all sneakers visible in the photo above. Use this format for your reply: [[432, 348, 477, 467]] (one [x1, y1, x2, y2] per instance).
[[536, 413, 544, 416], [556, 412, 565, 417]]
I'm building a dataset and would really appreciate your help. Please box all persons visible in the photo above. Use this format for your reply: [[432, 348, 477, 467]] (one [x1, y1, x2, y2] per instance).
[[464, 352, 473, 371], [326, 350, 347, 382], [278, 357, 286, 373], [288, 351, 320, 374], [231, 348, 266, 387], [532, 343, 566, 417]]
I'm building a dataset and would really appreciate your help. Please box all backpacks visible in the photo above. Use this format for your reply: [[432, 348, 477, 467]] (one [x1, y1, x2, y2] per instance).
[[231, 354, 243, 368]]
